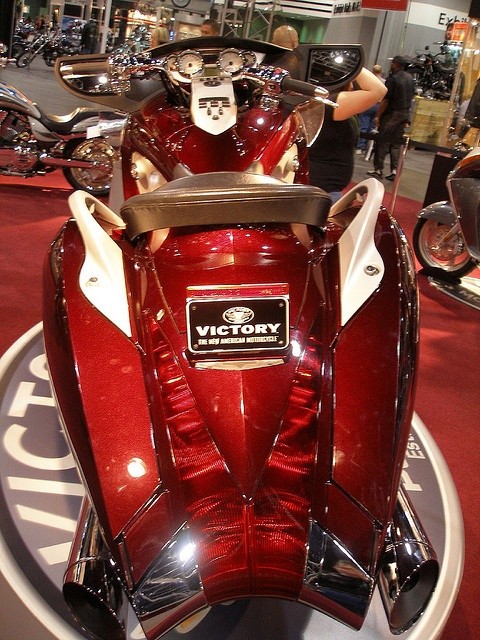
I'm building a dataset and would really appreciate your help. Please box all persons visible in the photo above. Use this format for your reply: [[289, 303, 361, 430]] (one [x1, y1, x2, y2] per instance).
[[271, 24, 299, 51], [201, 19, 221, 36], [24, 12, 100, 54], [354, 63, 388, 155], [367, 55, 417, 182], [150, 27, 170, 49], [306, 65, 389, 205]]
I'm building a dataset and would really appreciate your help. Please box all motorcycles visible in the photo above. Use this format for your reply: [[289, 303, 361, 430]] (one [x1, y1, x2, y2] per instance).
[[42, 35, 441, 640], [412, 117, 480, 281], [0, 82, 130, 197], [11, 17, 85, 68], [387, 41, 458, 101]]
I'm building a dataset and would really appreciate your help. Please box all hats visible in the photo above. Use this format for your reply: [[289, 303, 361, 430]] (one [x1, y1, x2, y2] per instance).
[[388, 56, 404, 65]]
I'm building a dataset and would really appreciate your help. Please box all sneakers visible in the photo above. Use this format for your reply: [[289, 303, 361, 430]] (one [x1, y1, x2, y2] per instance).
[[384, 173, 397, 182], [355, 149, 362, 155], [367, 169, 384, 179]]
